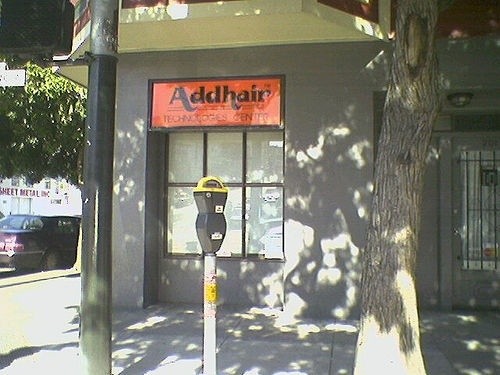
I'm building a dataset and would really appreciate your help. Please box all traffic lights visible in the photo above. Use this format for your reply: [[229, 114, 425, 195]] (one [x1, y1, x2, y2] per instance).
[[2, 0, 74, 56]]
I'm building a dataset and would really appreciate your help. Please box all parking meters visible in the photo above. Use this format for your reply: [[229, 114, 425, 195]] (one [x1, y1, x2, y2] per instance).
[[193, 176, 229, 374]]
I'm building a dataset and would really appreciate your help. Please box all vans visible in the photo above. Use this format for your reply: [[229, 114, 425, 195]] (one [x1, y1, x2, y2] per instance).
[[1, 215, 81, 272]]
